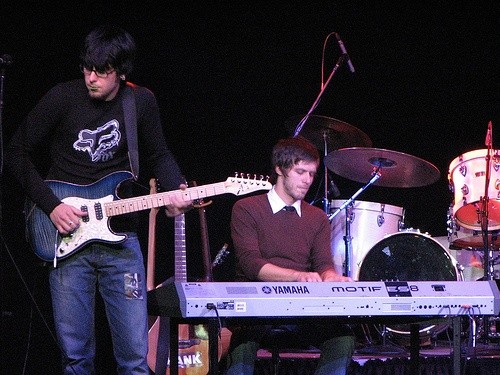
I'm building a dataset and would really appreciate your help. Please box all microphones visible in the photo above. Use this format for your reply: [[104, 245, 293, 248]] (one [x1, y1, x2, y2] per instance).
[[335, 33, 355, 72], [485, 122, 492, 146]]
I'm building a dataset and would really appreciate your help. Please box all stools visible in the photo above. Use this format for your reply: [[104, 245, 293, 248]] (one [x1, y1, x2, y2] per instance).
[[259, 328, 309, 375]]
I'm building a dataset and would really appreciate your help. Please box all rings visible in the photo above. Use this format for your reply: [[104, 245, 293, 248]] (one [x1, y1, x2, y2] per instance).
[[69, 222, 72, 225]]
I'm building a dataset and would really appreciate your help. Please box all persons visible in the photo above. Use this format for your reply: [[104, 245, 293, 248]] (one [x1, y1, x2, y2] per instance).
[[5, 26, 195, 375], [225, 135, 355, 375]]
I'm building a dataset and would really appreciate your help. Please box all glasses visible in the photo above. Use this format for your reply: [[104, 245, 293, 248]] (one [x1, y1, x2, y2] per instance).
[[79, 63, 116, 78]]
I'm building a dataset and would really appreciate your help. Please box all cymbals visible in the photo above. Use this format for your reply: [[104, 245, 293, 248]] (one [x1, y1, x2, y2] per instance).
[[323, 146, 443, 188], [282, 113, 373, 152]]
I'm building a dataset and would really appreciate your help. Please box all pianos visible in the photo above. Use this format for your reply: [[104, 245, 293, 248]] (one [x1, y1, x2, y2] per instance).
[[146, 278, 500, 375]]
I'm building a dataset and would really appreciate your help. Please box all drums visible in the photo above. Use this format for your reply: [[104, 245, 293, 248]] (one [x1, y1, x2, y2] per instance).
[[448, 147, 500, 232], [328, 198, 406, 282], [356, 230, 466, 338], [447, 201, 500, 252]]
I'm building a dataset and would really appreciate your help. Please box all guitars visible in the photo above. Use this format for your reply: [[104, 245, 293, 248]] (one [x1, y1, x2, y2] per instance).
[[147, 175, 233, 375], [24, 169, 274, 269]]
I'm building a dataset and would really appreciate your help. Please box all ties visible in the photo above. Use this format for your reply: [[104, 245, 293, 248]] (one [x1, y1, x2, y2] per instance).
[[284, 206, 295, 212]]
